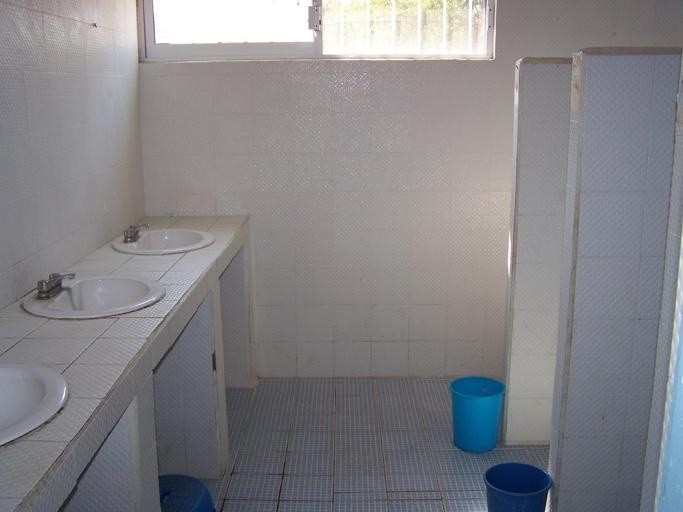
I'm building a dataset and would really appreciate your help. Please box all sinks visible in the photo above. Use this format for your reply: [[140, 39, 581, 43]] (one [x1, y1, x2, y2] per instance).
[[1, 357, 69, 447], [111, 226, 213, 256], [20, 273, 165, 320]]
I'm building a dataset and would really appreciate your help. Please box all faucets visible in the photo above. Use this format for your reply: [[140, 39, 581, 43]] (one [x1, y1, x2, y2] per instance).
[[37, 271, 75, 299], [123, 221, 150, 242]]
